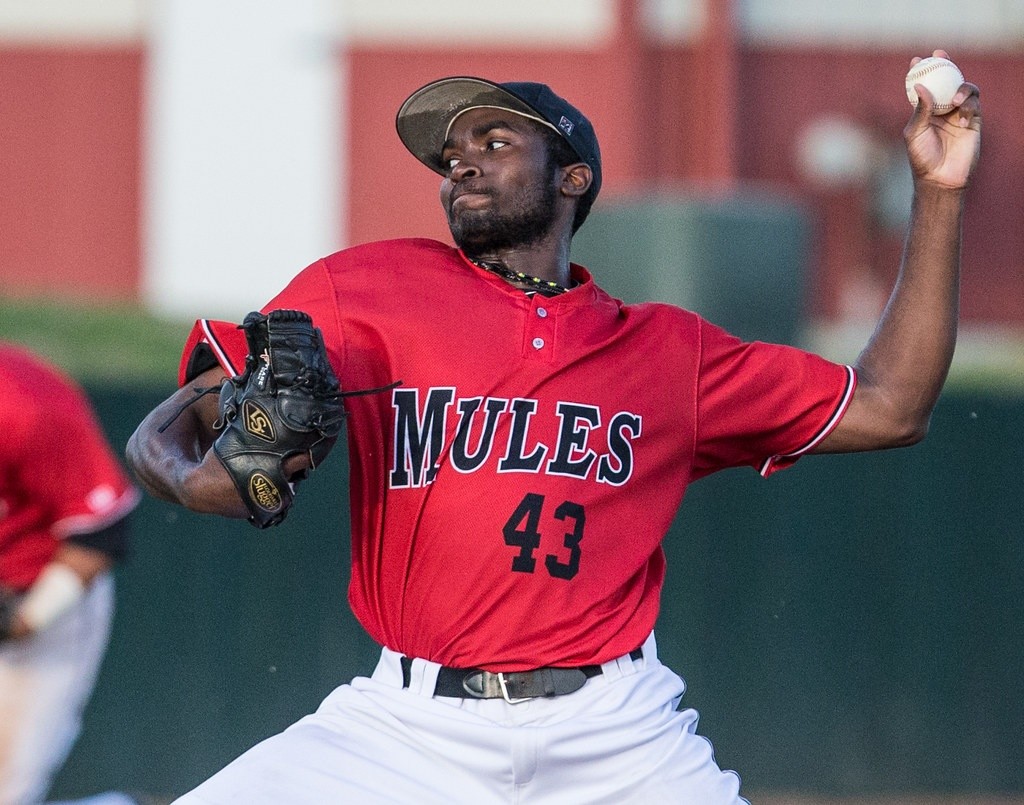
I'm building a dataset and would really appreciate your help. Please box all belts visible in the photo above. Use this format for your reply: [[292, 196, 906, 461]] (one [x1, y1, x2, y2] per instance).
[[401, 644, 644, 699]]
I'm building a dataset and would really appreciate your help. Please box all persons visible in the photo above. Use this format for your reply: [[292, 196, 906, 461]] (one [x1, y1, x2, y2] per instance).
[[114, 49, 987, 805], [2, 318, 140, 805]]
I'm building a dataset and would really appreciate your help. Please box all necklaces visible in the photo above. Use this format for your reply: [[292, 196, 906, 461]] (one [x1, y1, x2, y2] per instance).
[[472, 256, 575, 304]]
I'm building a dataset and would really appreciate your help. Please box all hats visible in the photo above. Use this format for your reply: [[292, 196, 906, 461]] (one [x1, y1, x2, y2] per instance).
[[394, 75, 603, 205]]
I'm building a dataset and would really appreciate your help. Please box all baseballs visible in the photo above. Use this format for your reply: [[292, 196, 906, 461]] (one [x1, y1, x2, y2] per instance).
[[904, 56, 964, 116]]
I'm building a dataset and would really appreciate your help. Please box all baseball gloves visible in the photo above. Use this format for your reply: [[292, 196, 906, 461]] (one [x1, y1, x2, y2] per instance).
[[214, 309, 346, 528]]
